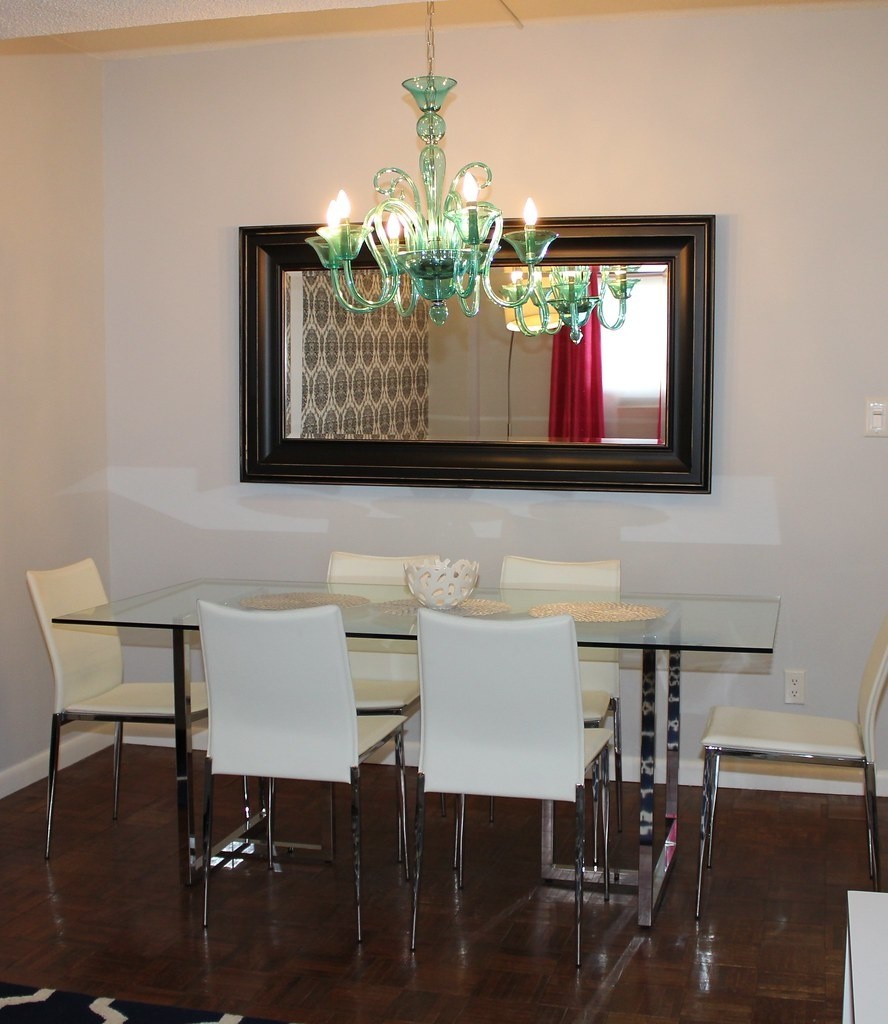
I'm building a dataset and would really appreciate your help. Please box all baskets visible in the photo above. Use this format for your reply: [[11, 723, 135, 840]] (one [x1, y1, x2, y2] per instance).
[[402, 556, 480, 614]]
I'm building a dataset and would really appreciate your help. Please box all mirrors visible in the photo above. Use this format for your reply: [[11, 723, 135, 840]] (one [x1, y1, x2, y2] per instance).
[[238, 215, 716, 496]]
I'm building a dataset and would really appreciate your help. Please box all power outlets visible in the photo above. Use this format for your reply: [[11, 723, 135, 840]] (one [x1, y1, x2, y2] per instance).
[[781, 669, 805, 704]]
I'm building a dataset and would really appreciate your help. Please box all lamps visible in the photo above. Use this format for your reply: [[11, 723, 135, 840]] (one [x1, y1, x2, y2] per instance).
[[500, 263, 642, 344], [303, 0, 560, 327], [503, 265, 567, 333]]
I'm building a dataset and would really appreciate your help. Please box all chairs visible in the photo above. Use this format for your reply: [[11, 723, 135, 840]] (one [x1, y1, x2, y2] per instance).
[[498, 555, 623, 837], [25, 557, 251, 862], [416, 603, 615, 970], [197, 596, 412, 945], [691, 609, 888, 921], [315, 548, 474, 863]]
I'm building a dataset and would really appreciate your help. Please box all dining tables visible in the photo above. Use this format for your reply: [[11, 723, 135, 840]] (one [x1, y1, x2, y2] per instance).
[[49, 577, 783, 931]]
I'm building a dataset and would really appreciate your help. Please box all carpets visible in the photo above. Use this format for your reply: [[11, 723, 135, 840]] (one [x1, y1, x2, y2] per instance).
[[0, 981, 306, 1024]]
